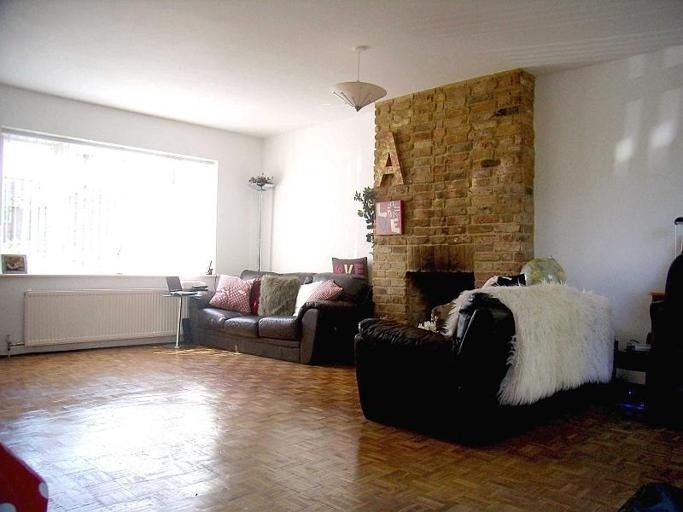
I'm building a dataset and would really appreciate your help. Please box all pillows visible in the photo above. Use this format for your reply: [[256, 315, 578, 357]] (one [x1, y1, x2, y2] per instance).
[[209, 257, 368, 317]]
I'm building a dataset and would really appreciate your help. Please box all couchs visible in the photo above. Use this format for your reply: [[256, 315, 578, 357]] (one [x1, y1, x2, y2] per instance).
[[187, 270, 372, 365], [355, 283, 616, 449]]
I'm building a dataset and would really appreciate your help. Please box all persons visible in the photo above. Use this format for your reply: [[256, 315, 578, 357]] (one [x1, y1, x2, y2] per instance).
[[3, 256, 23, 271]]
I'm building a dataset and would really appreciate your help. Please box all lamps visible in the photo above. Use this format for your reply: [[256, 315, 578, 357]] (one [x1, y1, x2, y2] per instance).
[[329, 44, 387, 112]]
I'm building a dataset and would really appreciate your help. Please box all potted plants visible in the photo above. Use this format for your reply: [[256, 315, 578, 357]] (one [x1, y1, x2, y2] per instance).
[[204, 260, 216, 288]]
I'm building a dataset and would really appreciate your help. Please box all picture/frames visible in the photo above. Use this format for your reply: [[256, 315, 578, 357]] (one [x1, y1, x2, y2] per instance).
[[0, 253, 28, 275]]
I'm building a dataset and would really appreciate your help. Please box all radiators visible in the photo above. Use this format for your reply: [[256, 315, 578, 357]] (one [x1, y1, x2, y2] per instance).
[[24, 288, 188, 348]]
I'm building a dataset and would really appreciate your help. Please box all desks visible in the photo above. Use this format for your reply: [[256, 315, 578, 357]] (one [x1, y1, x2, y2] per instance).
[[161, 294, 203, 349]]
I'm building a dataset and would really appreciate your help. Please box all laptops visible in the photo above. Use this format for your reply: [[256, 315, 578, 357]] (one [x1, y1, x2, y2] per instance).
[[166, 276, 198, 294]]
